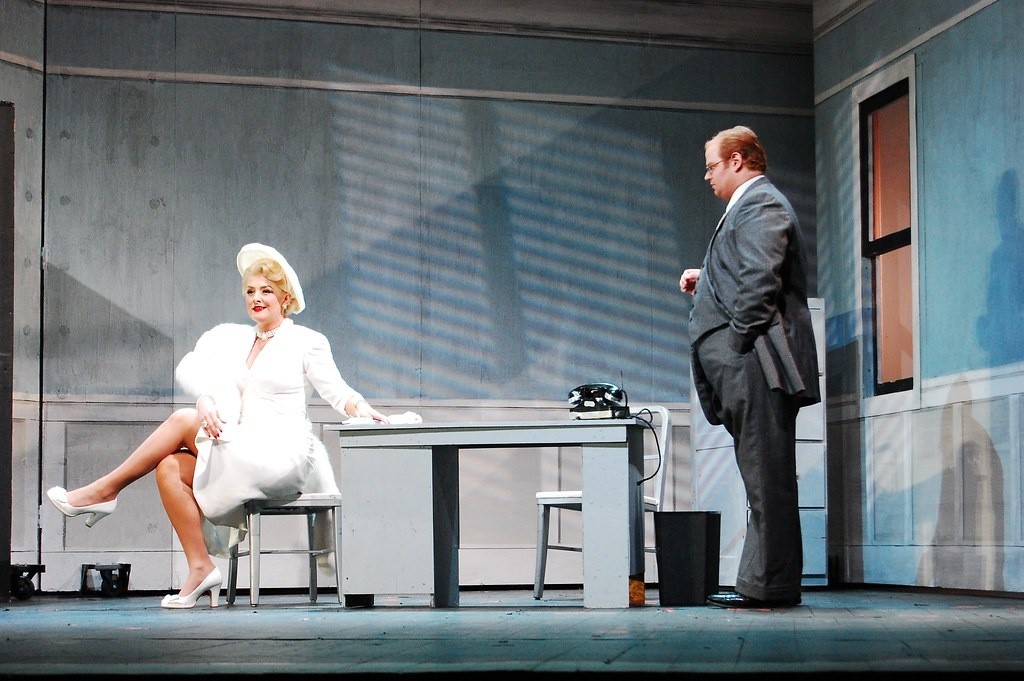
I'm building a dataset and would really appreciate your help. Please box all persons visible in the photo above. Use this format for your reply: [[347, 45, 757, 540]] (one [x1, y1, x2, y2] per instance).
[[679, 125, 823, 610], [46, 243, 391, 609]]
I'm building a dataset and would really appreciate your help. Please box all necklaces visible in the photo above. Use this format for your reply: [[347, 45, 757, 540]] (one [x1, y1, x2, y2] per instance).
[[256, 326, 279, 342]]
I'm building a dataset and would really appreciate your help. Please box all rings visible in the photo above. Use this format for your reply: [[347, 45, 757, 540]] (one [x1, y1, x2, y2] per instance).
[[202, 421, 209, 428]]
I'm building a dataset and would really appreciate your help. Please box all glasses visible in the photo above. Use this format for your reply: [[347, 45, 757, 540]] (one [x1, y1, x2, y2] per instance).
[[706, 160, 722, 170]]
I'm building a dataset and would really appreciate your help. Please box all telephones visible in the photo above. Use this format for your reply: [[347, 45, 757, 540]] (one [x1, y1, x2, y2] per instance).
[[568, 382, 630, 420]]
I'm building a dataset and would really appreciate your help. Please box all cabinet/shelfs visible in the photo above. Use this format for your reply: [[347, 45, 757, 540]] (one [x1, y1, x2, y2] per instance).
[[689, 297, 828, 591]]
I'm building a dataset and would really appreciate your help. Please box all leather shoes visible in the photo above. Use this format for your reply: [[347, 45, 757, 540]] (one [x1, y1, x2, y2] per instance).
[[706, 591, 802, 608]]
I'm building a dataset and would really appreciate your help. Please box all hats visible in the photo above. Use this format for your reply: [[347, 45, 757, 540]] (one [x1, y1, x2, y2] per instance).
[[236, 243, 306, 315]]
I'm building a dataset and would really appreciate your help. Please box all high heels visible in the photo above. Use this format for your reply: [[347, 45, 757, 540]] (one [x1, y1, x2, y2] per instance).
[[46, 486, 118, 529], [161, 566, 223, 609]]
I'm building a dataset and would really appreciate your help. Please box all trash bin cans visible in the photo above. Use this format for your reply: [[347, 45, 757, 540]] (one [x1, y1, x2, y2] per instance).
[[652, 510, 722, 608]]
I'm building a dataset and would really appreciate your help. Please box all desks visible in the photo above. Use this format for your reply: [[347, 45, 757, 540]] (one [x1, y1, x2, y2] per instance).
[[323, 419, 657, 610]]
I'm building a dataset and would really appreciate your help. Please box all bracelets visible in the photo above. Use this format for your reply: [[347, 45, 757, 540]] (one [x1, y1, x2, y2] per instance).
[[196, 394, 216, 409], [354, 399, 366, 411]]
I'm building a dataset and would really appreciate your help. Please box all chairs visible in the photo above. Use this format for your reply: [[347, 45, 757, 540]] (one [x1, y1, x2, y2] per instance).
[[533, 403, 672, 601], [226, 492, 342, 608]]
